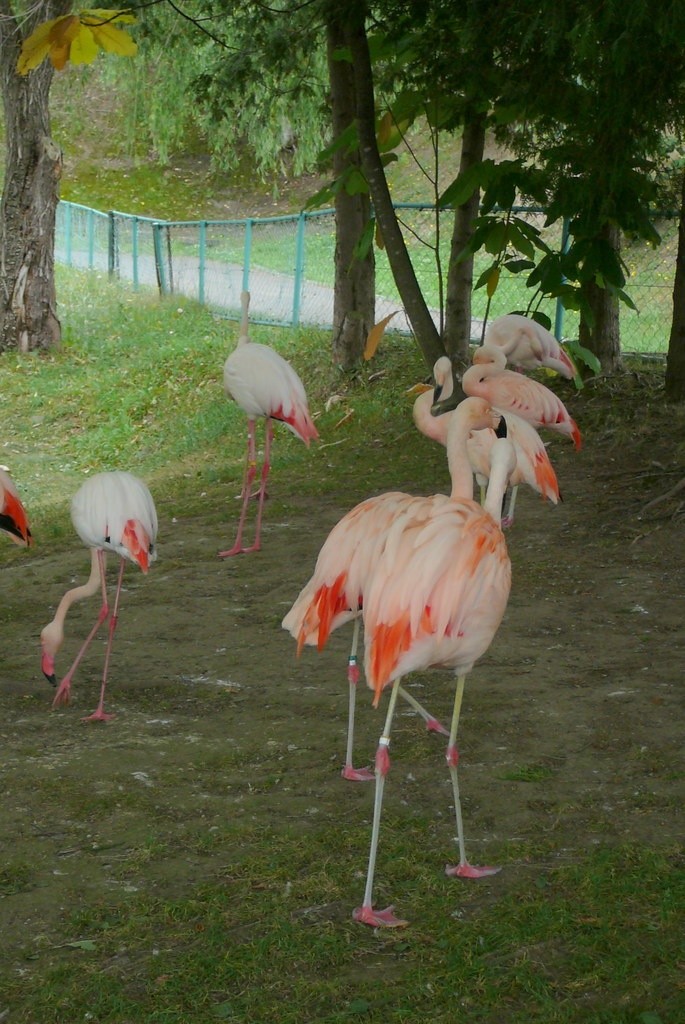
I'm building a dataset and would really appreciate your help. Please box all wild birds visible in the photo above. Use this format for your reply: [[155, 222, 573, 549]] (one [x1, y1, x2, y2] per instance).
[[216, 291, 322, 559], [0, 466, 34, 552], [350, 437, 519, 929], [280, 396, 510, 783], [40, 470, 158, 720], [413, 314, 583, 531]]
